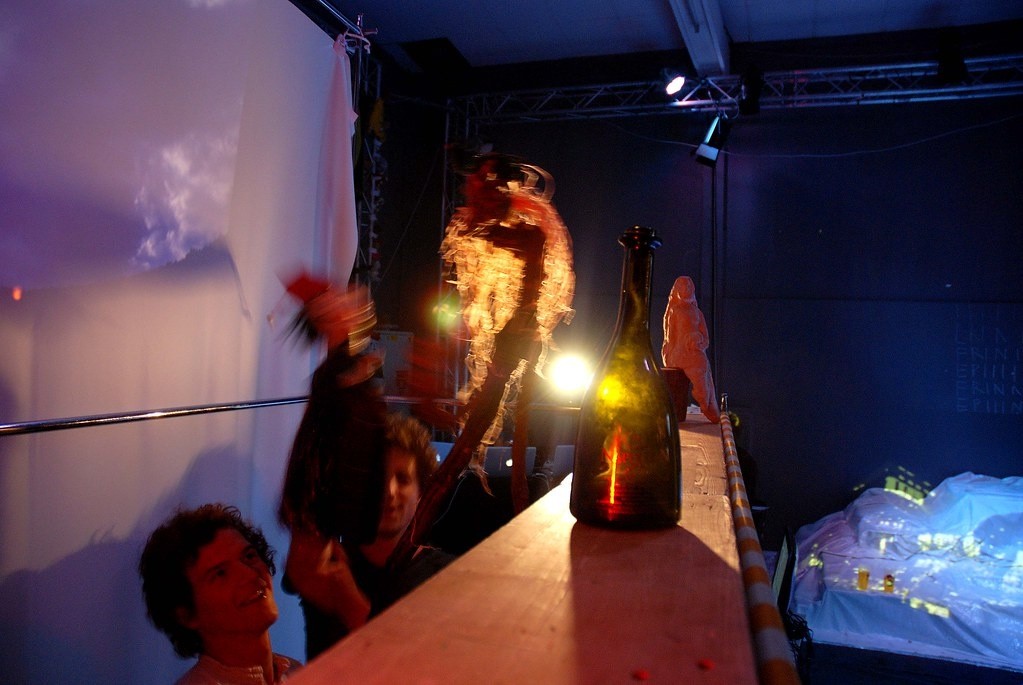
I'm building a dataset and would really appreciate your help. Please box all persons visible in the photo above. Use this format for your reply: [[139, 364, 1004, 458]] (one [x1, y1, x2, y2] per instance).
[[661, 276, 720, 424], [140, 270, 543, 685]]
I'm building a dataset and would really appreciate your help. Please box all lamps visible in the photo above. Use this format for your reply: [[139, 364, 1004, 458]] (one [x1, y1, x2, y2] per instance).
[[656, 67, 684, 98]]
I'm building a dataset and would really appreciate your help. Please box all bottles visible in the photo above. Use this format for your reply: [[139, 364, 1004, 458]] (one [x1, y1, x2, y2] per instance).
[[568, 222, 683, 533]]
